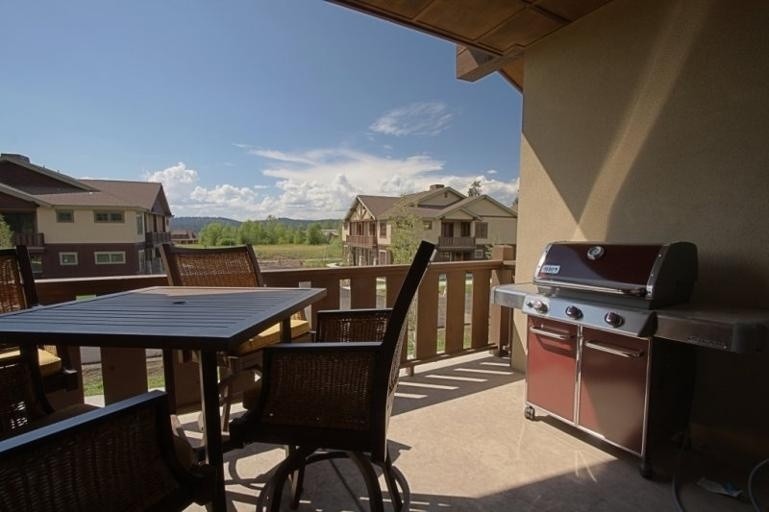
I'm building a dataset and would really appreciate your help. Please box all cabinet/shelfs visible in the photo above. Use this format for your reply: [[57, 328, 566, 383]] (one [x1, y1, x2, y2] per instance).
[[524, 315, 700, 460]]
